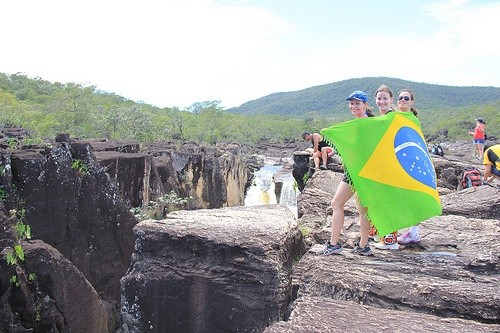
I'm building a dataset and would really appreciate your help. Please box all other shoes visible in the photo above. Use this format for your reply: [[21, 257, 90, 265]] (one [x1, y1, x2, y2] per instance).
[[320, 165, 327, 169], [309, 166, 316, 172]]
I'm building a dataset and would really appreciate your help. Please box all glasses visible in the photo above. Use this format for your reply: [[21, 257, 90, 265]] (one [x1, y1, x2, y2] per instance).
[[303, 136, 305, 139], [398, 95, 410, 100]]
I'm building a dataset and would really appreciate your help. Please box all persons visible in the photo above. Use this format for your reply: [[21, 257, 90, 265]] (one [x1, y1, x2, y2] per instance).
[[467, 118, 486, 159], [300, 130, 336, 172], [315, 90, 375, 257], [396, 88, 422, 245], [373, 84, 400, 251], [482, 141, 500, 182]]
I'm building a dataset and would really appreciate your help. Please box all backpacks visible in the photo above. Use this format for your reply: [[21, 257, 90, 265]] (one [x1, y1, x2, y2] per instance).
[[461, 167, 483, 189]]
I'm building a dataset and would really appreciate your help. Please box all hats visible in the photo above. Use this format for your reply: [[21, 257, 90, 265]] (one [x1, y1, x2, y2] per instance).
[[475, 118, 483, 121], [346, 90, 367, 102]]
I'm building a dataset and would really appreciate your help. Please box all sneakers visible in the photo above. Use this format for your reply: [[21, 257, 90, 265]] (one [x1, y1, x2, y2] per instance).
[[367, 224, 380, 239], [350, 241, 372, 255], [316, 239, 342, 254], [373, 241, 399, 249], [397, 231, 420, 243]]
